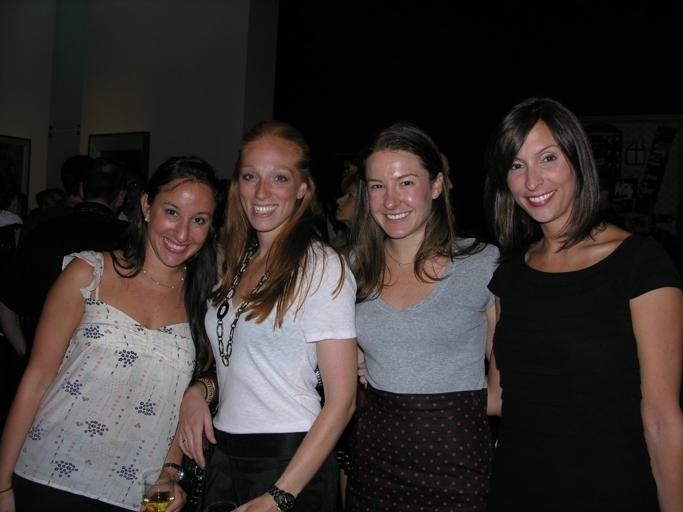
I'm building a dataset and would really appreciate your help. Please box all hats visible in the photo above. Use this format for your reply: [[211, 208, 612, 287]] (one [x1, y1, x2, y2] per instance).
[[0, 209, 24, 228]]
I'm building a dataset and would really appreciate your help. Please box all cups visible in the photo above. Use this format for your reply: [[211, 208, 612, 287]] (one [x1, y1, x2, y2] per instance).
[[142, 469, 176, 512]]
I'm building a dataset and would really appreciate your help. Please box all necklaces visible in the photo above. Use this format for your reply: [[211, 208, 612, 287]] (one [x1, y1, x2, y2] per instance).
[[384, 244, 426, 266], [215, 240, 270, 367], [139, 262, 187, 289]]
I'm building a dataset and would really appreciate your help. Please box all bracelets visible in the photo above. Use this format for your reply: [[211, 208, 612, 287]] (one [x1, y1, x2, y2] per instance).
[[162, 462, 181, 473], [197, 375, 218, 405], [0, 486, 13, 494]]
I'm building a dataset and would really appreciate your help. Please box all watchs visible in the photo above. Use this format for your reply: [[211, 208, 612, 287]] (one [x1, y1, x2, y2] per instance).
[[264, 484, 297, 512]]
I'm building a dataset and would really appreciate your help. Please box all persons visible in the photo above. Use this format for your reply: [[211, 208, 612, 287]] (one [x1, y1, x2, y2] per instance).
[[329, 168, 368, 254], [174, 119, 361, 512], [340, 121, 504, 511], [356, 93, 683, 512], [0, 153, 217, 512]]
